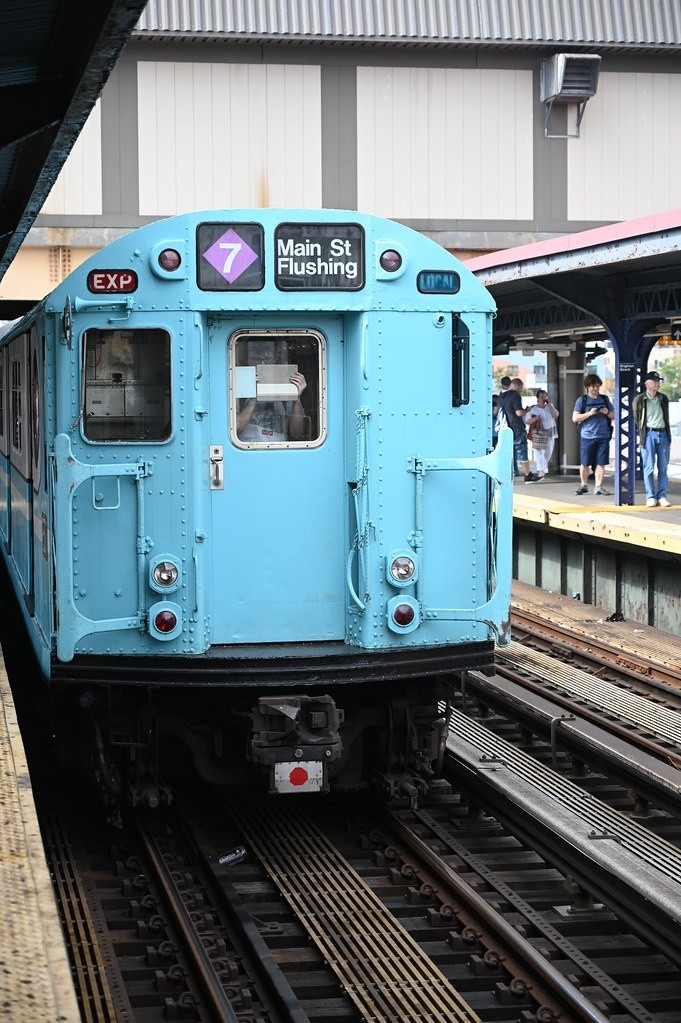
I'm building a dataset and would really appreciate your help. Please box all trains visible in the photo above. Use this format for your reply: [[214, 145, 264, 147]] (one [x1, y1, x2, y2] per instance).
[[0, 206, 515, 817]]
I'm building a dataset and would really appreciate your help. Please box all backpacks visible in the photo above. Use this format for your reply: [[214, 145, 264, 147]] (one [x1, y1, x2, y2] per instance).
[[494, 390, 516, 434]]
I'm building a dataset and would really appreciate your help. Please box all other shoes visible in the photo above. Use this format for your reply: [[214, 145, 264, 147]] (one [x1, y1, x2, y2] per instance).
[[657, 497, 671, 507], [646, 498, 657, 506]]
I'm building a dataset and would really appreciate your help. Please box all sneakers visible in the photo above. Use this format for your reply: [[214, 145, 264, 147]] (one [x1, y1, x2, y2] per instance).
[[524, 472, 544, 485], [575, 485, 588, 494], [594, 486, 610, 495]]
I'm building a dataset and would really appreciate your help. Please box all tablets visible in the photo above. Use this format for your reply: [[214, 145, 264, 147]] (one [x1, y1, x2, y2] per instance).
[[257, 364, 299, 402]]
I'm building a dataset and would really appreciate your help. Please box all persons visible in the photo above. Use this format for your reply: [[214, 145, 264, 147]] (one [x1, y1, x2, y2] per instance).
[[524, 389, 560, 480], [233, 341, 309, 447], [572, 373, 616, 496], [631, 371, 671, 508], [491, 376, 544, 486]]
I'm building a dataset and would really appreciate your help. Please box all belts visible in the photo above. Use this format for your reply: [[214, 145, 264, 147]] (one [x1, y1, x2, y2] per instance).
[[646, 428, 666, 433]]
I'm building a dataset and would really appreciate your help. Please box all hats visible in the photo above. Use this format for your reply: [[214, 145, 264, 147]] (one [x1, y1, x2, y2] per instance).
[[644, 371, 664, 382]]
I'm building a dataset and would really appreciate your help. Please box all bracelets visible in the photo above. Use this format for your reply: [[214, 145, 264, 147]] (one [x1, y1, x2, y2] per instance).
[[605, 410, 609, 415]]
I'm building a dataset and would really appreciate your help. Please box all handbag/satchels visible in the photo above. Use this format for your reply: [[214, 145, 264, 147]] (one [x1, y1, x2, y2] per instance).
[[531, 418, 549, 449]]
